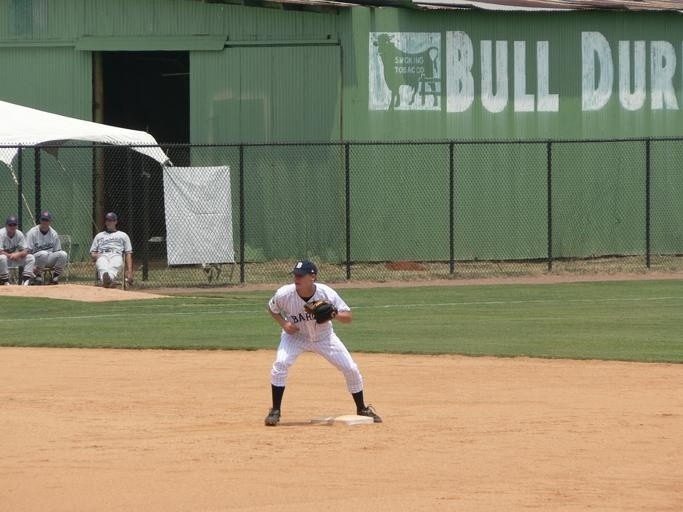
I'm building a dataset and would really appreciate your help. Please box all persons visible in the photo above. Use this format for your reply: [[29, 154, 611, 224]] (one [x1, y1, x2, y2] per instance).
[[24, 212, 68, 285], [265, 260, 383, 426], [0, 217, 35, 285], [90, 213, 134, 288]]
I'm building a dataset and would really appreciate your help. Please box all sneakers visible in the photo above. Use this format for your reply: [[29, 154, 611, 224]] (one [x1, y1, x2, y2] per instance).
[[361, 407, 383, 422], [265, 409, 280, 425]]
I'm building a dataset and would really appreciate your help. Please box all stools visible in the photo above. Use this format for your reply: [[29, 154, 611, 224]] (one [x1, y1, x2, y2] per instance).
[[36, 268, 53, 284], [6, 267, 18, 283]]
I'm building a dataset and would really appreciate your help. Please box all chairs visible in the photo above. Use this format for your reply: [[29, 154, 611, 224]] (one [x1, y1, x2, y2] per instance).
[[96, 249, 126, 289], [57, 234, 72, 284]]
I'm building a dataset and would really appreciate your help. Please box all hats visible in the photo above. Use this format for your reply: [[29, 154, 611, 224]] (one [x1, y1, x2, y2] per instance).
[[289, 260, 317, 275], [105, 212, 117, 220], [7, 216, 17, 225], [40, 212, 51, 220]]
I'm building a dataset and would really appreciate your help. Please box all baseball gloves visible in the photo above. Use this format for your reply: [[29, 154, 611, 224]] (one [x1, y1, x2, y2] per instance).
[[303, 299, 338, 324]]
